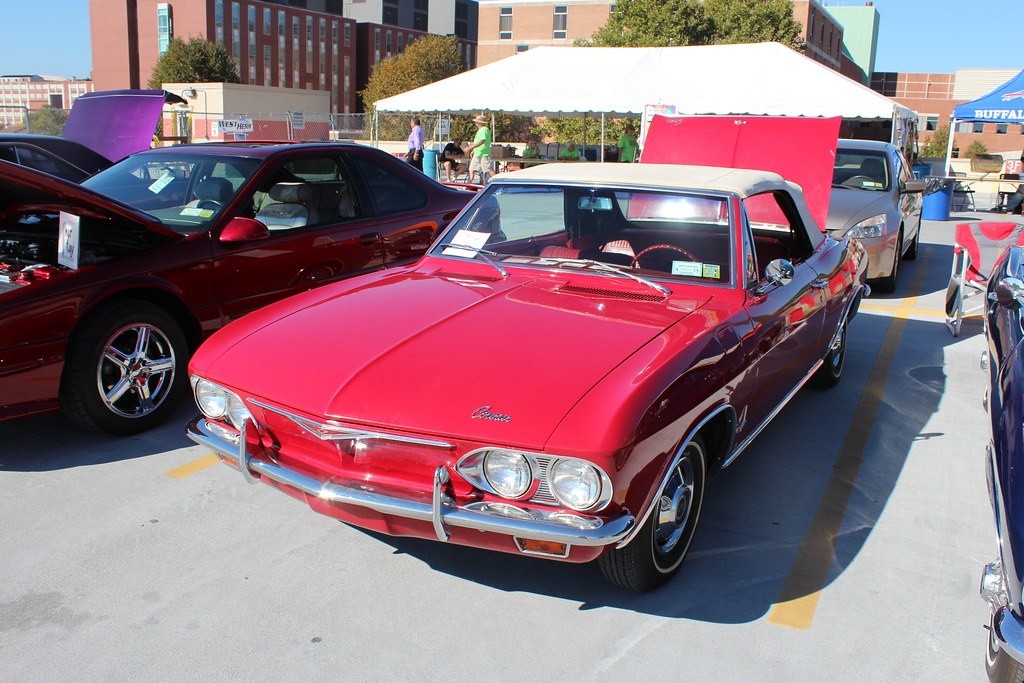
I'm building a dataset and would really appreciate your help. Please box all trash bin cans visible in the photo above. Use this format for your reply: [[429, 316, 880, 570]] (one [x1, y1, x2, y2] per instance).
[[911, 162, 932, 180], [921, 176, 956, 221], [422, 148, 440, 180], [951, 148, 959, 158]]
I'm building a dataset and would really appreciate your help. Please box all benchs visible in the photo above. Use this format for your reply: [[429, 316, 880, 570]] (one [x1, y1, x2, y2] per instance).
[[251, 180, 359, 222], [592, 227, 792, 292]]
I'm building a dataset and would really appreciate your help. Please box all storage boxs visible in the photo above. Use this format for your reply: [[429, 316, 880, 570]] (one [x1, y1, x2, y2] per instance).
[[489, 147, 510, 159]]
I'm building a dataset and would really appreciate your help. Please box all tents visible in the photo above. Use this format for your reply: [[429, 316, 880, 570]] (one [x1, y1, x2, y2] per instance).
[[373, 42, 919, 163], [944, 68, 1024, 177]]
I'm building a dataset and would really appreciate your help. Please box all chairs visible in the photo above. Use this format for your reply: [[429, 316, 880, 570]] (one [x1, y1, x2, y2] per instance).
[[185, 178, 236, 217], [252, 182, 320, 230], [843, 158, 887, 193], [997, 173, 1019, 213], [949, 172, 976, 212], [435, 153, 456, 183]]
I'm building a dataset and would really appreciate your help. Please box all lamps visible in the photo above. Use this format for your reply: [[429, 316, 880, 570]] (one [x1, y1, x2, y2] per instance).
[[185, 88, 195, 97]]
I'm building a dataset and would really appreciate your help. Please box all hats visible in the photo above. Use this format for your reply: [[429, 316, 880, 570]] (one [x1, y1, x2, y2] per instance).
[[472, 115, 489, 123], [567, 141, 574, 147]]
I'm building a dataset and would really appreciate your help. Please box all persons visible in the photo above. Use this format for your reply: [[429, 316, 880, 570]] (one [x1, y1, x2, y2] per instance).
[[464, 115, 496, 184], [558, 141, 580, 161], [438, 138, 468, 183], [523, 138, 541, 168], [617, 124, 640, 163], [404, 118, 424, 173]]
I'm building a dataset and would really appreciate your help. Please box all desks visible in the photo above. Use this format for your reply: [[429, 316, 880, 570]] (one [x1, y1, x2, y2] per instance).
[[457, 158, 579, 187], [924, 176, 1024, 217]]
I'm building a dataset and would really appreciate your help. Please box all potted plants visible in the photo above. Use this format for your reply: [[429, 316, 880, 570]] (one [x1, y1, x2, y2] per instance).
[[918, 126, 950, 177]]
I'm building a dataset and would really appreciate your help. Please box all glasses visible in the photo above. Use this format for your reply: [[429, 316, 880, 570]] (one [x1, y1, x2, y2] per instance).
[[630, 128, 635, 130]]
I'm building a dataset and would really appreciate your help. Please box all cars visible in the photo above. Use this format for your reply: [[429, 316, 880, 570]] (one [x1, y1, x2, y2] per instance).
[[0, 139, 509, 441], [183, 161, 870, 594], [980, 242, 1024, 683], [825, 138, 927, 295], [0, 88, 192, 204]]
